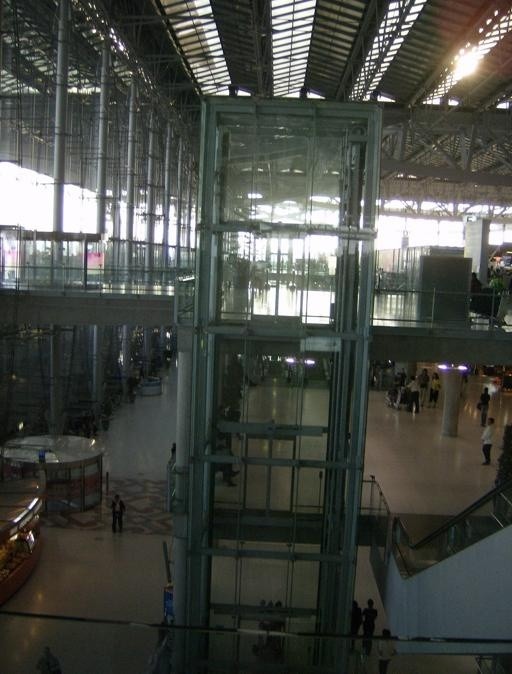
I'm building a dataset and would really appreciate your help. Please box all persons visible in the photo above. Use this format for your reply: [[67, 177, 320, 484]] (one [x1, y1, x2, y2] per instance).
[[352, 597, 377, 655], [479, 387, 491, 425], [215, 430, 239, 487], [109, 494, 126, 534], [471, 272, 481, 312], [384, 367, 441, 413], [377, 627, 399, 674], [35, 645, 62, 674], [65, 396, 115, 438], [256, 599, 283, 654], [479, 417, 495, 466]]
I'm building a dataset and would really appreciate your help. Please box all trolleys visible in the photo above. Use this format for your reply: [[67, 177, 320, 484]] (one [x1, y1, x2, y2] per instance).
[[384, 387, 409, 409]]
